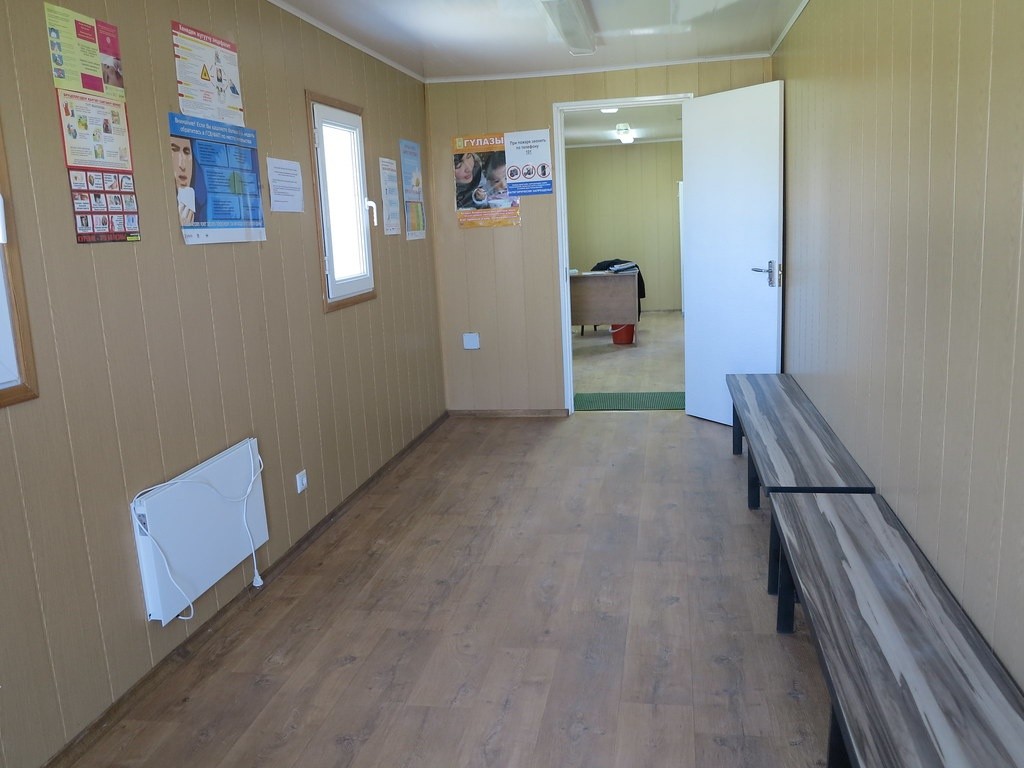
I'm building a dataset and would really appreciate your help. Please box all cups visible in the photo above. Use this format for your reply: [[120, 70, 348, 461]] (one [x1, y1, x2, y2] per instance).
[[569, 269, 578, 276]]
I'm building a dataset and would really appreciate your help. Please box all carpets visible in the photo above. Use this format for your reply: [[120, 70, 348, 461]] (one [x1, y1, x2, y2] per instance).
[[574, 392, 685, 411]]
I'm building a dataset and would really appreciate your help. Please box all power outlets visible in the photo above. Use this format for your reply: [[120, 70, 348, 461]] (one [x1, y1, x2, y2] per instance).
[[296, 469, 308, 494]]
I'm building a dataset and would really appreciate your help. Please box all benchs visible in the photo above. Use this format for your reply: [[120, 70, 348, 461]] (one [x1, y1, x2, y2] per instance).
[[766, 491, 1024, 768], [726, 374, 876, 595]]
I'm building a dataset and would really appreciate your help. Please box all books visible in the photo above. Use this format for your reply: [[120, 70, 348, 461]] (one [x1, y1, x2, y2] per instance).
[[605, 262, 638, 274]]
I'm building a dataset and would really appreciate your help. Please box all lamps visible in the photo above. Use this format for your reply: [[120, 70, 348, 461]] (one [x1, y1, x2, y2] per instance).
[[539, 0, 599, 57], [617, 123, 634, 144]]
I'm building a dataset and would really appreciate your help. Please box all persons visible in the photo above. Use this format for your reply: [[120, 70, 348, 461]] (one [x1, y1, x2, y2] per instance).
[[111, 216, 114, 231], [454, 154, 484, 210], [81, 216, 88, 227], [102, 216, 107, 225], [93, 194, 104, 206], [472, 152, 519, 208], [102, 58, 124, 88]]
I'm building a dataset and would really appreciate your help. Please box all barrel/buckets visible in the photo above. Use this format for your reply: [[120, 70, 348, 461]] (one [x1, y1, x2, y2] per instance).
[[609, 323, 634, 344]]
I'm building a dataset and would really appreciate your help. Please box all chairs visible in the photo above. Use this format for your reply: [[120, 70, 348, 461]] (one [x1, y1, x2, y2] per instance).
[[581, 259, 645, 335]]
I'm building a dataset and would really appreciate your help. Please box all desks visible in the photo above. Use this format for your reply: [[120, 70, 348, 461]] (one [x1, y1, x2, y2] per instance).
[[570, 268, 639, 347]]
